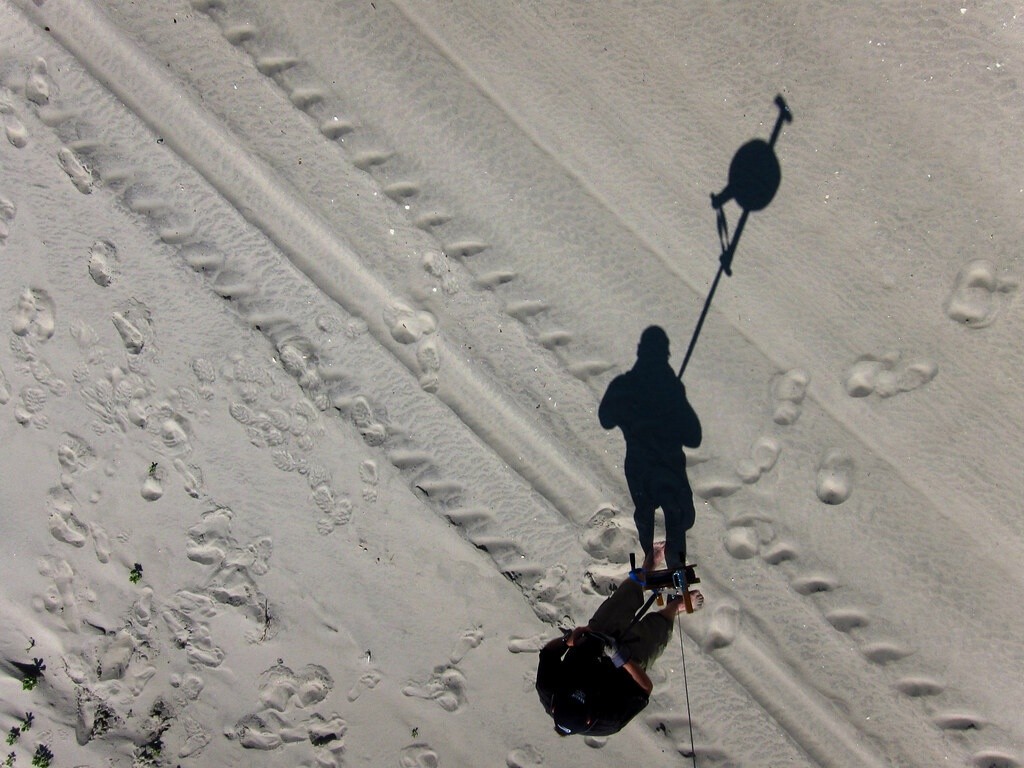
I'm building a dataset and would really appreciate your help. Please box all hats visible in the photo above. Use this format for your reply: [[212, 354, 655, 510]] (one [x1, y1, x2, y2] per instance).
[[553, 690, 590, 738]]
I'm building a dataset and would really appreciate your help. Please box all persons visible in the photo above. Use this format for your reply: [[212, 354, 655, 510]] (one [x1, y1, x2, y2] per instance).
[[529, 544, 705, 736]]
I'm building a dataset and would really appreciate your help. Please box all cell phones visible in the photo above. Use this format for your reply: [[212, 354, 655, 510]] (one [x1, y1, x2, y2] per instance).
[[586, 633, 606, 649]]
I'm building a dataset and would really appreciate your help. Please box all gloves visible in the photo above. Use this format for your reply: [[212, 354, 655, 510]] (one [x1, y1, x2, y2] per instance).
[[596, 631, 629, 669]]
